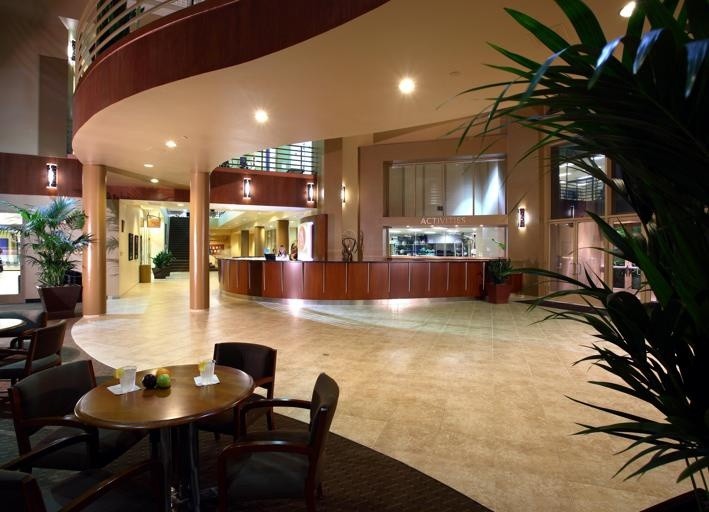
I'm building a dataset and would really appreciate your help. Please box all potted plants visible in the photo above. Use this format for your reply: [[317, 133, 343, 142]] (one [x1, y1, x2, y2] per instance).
[[152, 250, 170, 279], [161, 252, 176, 276], [517, 208, 526, 228], [485, 258, 518, 305], [2, 191, 99, 320], [454, 0, 707, 510]]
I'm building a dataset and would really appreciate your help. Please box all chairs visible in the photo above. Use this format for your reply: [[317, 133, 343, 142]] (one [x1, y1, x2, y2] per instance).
[[0, 361, 163, 512], [214, 371, 341, 508], [0, 310, 68, 409], [191, 341, 279, 436]]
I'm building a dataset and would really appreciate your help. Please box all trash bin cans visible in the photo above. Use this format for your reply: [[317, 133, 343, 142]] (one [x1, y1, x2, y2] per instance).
[[140, 265, 151, 283]]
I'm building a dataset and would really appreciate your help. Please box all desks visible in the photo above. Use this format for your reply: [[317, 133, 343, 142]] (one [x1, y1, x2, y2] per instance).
[[77, 360, 253, 510]]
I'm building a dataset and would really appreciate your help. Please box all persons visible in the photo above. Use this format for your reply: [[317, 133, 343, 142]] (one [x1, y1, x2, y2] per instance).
[[278, 244, 289, 261], [298, 226, 307, 260], [0, 248, 5, 273], [290, 242, 297, 260], [426, 245, 435, 255]]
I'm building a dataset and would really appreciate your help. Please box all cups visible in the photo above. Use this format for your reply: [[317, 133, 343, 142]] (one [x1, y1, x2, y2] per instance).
[[199, 359, 216, 385], [117, 364, 137, 391]]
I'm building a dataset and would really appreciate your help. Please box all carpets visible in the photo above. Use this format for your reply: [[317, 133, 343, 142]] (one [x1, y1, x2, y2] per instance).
[[0, 312, 495, 511]]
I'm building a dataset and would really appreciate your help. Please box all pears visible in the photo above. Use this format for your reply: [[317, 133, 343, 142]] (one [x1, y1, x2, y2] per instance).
[[156, 374, 171, 389]]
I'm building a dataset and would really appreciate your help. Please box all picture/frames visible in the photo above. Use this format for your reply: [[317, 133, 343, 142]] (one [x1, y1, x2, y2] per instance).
[[134, 234, 138, 260], [128, 232, 133, 260]]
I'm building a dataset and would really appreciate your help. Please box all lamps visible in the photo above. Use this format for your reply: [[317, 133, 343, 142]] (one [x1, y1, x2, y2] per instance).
[[45, 162, 58, 191], [340, 186, 346, 202], [307, 182, 316, 203], [242, 176, 252, 200]]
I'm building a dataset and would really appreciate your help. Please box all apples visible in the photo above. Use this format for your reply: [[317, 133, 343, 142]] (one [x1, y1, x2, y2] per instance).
[[142, 374, 156, 388]]
